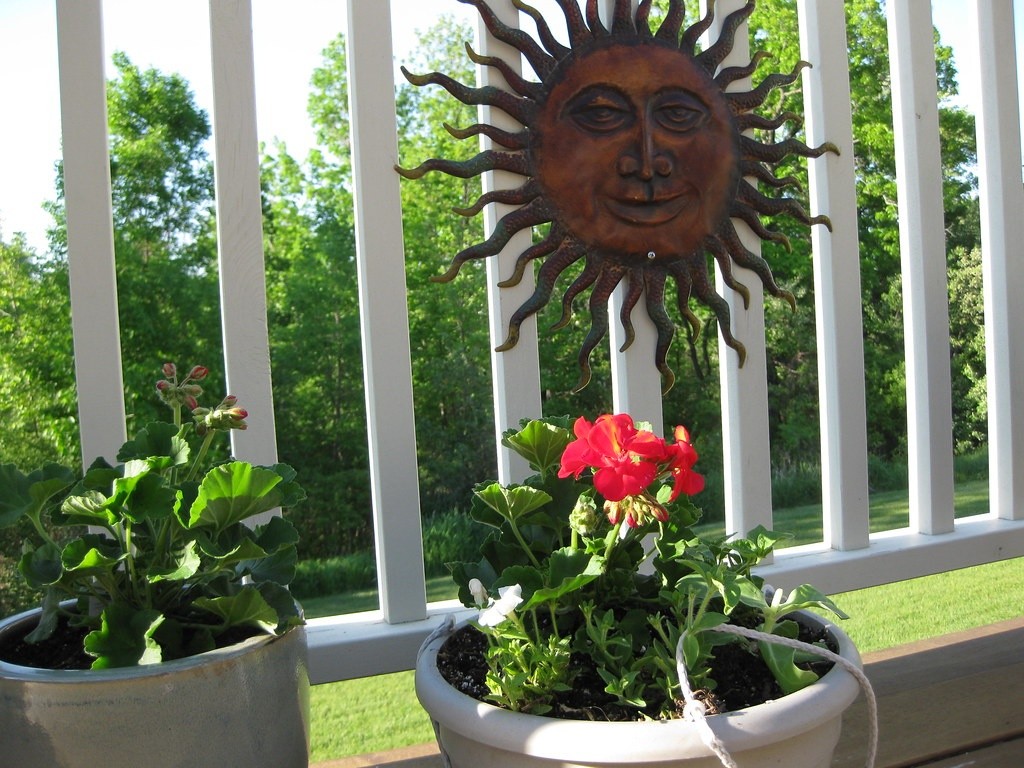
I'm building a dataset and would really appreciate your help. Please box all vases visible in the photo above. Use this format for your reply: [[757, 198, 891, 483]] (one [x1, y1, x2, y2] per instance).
[[1, 584, 312, 768], [416, 601, 867, 768]]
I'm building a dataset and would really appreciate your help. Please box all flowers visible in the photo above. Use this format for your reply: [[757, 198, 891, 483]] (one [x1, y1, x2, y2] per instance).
[[441, 413, 852, 721], [1, 363, 306, 668]]
